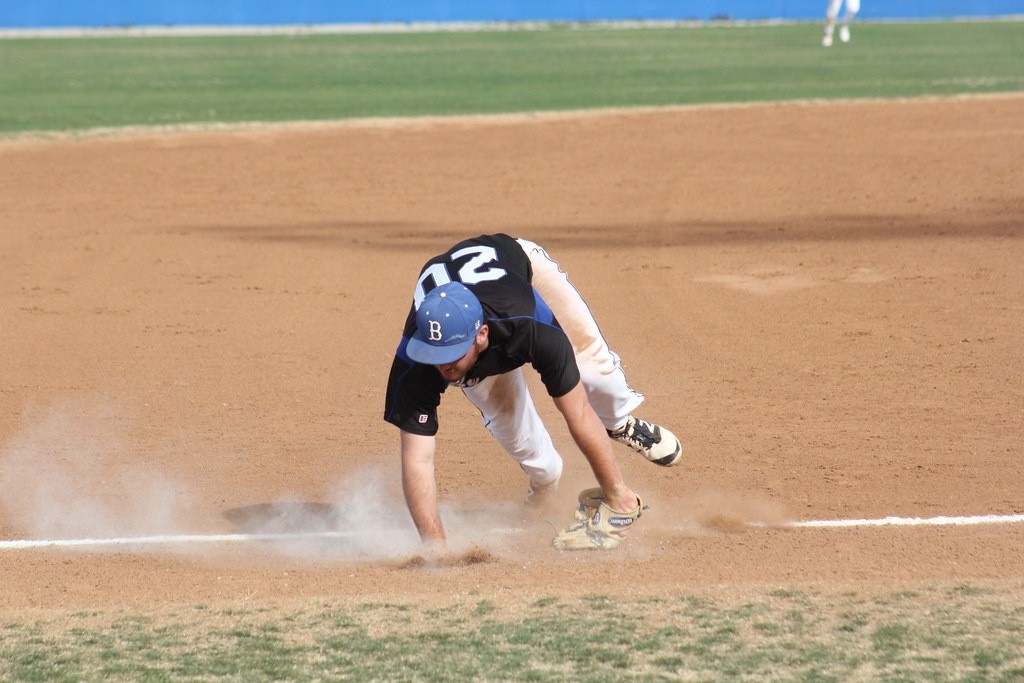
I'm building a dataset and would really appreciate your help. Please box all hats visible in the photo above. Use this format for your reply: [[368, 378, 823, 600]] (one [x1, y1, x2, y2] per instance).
[[405, 281, 485, 364]]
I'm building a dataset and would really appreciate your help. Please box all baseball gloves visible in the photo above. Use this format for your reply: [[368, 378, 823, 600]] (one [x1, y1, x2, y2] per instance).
[[551, 487, 644, 553]]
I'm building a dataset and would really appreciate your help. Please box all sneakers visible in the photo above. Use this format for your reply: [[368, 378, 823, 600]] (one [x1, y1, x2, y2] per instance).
[[525, 476, 560, 517], [606, 414, 682, 467]]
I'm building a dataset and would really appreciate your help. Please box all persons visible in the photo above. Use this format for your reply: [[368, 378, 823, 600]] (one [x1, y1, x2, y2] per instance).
[[383, 233, 682, 554], [821, 0, 860, 47]]
